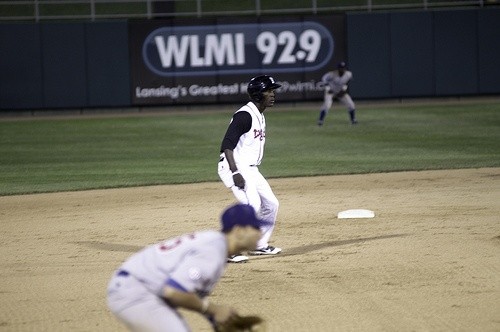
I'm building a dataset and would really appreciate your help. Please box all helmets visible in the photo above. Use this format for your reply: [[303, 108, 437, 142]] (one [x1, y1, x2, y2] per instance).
[[247, 74, 282, 97]]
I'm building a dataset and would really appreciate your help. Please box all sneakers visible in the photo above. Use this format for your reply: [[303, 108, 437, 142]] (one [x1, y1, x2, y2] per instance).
[[248, 246, 282, 256], [226, 253, 249, 264]]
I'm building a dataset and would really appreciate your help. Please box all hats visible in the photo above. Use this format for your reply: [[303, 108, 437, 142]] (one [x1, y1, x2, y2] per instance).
[[219, 204, 273, 231], [337, 62, 348, 68]]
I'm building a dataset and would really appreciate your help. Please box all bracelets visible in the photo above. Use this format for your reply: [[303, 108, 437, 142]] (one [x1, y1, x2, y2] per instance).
[[201, 302, 208, 312], [232, 170, 239, 175]]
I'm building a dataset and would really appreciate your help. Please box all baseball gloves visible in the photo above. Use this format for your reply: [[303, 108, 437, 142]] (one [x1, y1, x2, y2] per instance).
[[206, 312, 266, 332]]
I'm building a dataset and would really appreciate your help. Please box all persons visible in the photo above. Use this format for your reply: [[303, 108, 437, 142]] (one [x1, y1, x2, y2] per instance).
[[318, 61, 357, 126], [218, 75, 281, 262], [106, 204, 273, 332]]
